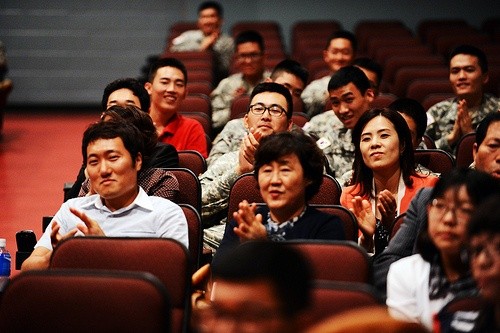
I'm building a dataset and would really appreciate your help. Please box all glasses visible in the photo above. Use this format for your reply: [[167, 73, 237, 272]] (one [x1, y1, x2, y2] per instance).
[[250, 103, 287, 117]]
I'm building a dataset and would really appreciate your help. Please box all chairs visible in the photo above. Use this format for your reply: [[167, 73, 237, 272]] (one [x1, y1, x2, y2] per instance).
[[0, 20, 500, 333]]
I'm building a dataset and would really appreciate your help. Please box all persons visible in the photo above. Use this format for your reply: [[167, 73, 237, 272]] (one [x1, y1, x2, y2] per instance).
[[302, 34, 441, 190], [208, 130, 350, 274], [424, 49, 500, 162], [170, 1, 235, 73], [338, 109, 438, 258], [369, 110, 500, 292], [67, 58, 208, 202], [21, 119, 188, 278], [197, 82, 294, 259], [198, 31, 313, 261], [200, 241, 316, 332], [433, 202, 500, 332]]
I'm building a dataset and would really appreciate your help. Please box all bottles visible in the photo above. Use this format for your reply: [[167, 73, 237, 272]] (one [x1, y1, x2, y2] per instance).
[[0, 238, 11, 291]]
